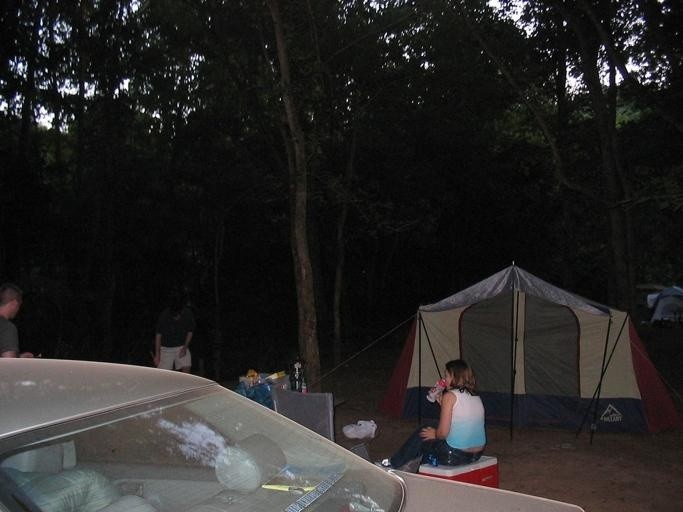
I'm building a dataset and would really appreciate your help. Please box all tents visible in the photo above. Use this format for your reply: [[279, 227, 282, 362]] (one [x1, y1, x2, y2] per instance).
[[378, 263, 683, 435], [650, 285, 682, 325]]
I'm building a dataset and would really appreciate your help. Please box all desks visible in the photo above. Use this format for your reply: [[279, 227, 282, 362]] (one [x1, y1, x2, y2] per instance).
[[235, 373, 292, 414]]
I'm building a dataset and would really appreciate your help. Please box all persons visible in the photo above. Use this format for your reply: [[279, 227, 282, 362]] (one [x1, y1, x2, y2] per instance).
[[374, 360, 487, 469], [0, 283, 35, 358], [153, 300, 195, 374]]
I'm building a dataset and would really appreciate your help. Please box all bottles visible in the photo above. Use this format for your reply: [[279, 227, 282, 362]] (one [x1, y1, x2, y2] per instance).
[[291, 367, 302, 392], [425, 378, 447, 403]]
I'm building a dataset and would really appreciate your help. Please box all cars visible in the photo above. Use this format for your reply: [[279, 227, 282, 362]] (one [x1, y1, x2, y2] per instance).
[[0, 353, 580, 512]]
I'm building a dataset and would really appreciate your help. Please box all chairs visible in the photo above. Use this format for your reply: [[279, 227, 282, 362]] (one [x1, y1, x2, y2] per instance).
[[264, 384, 338, 444], [0, 430, 287, 512], [262, 375, 291, 394]]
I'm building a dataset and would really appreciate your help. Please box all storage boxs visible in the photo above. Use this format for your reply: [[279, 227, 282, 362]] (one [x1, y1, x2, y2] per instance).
[[416, 453, 500, 492]]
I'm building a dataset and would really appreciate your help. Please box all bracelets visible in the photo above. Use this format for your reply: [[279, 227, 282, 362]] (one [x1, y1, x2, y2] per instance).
[[435, 428, 438, 439]]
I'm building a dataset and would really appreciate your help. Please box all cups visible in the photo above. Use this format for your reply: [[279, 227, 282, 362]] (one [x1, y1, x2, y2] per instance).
[[247, 374, 262, 390]]
